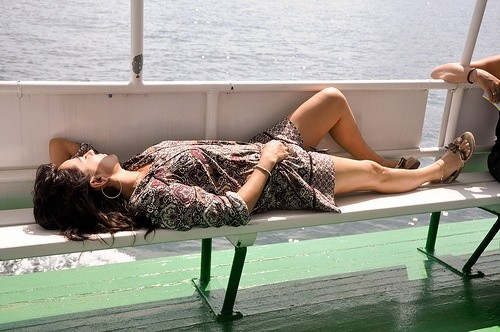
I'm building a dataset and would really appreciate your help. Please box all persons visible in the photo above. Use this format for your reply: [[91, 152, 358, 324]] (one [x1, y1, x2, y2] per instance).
[[33, 87, 475, 232], [431, 54, 500, 184]]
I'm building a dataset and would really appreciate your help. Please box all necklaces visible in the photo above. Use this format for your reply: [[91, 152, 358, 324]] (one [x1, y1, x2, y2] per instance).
[[135, 169, 144, 187]]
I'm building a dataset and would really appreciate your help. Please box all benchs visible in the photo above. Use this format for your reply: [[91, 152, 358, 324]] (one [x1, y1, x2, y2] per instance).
[[0, 171, 500, 321]]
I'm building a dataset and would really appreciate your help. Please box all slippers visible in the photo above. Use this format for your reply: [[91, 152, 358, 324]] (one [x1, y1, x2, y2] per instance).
[[443, 131, 475, 183], [395, 156, 420, 169]]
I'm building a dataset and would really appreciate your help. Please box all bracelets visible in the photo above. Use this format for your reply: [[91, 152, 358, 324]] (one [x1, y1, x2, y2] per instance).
[[253, 165, 272, 177], [467, 67, 476, 84]]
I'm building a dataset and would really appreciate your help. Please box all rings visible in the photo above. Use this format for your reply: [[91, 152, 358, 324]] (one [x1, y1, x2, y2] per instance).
[[492, 92, 497, 95]]
